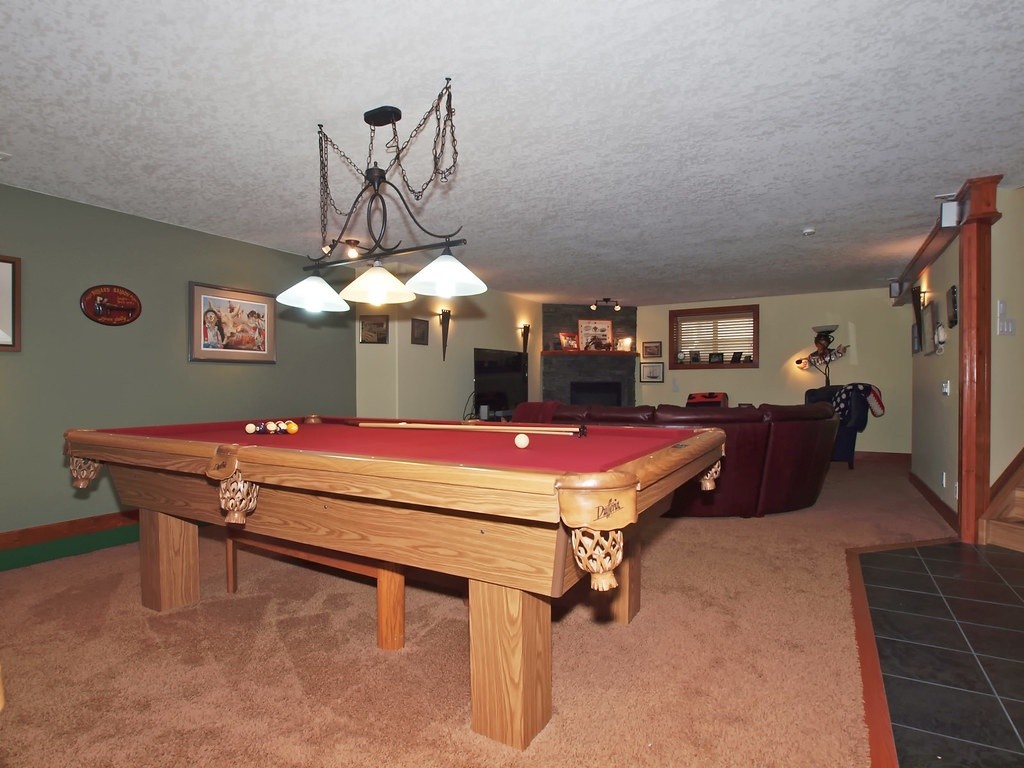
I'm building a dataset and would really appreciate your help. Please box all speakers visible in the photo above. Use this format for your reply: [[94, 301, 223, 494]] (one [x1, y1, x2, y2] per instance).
[[480, 405, 489, 420]]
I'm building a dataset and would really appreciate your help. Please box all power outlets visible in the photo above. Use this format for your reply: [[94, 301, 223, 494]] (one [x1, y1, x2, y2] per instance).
[[942, 472, 946, 488], [941, 380, 950, 397], [953, 482, 958, 500]]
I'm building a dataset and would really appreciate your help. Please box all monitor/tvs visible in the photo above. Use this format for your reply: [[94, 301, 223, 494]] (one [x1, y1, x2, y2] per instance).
[[473, 347, 528, 419]]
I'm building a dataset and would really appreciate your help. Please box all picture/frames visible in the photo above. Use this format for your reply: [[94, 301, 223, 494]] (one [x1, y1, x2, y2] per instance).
[[690, 351, 743, 363], [560, 319, 634, 351], [911, 323, 920, 354], [411, 318, 428, 346], [921, 299, 937, 357], [359, 314, 389, 343], [0, 253, 24, 354], [946, 285, 958, 329], [639, 362, 664, 383], [642, 341, 663, 358], [187, 279, 278, 365]]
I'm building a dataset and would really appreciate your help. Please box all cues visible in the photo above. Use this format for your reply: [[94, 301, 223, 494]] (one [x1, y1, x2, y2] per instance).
[[358, 422, 586, 438]]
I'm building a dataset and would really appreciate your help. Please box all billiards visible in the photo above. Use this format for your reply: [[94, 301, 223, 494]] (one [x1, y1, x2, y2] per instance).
[[515, 434, 529, 449], [245, 423, 256, 433], [277, 423, 287, 434], [266, 420, 293, 424], [287, 423, 299, 434], [265, 423, 276, 434], [256, 423, 265, 433]]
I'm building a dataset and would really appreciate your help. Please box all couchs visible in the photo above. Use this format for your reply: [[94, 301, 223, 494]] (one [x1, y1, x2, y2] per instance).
[[805, 384, 862, 470], [552, 399, 840, 519]]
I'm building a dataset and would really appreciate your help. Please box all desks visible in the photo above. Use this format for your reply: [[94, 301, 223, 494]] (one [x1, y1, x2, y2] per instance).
[[64, 413, 728, 752]]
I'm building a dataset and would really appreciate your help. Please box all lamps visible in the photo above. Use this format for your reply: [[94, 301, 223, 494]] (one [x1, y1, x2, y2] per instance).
[[910, 286, 926, 352], [519, 324, 529, 353], [889, 281, 901, 298], [438, 308, 451, 361], [934, 322, 947, 355], [274, 78, 487, 314], [590, 298, 621, 311]]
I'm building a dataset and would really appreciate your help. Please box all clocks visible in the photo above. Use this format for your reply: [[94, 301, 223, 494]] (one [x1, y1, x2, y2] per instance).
[[676, 350, 685, 364]]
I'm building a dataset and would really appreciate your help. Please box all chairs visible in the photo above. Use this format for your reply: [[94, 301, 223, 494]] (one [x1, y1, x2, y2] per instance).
[[511, 401, 562, 422]]
